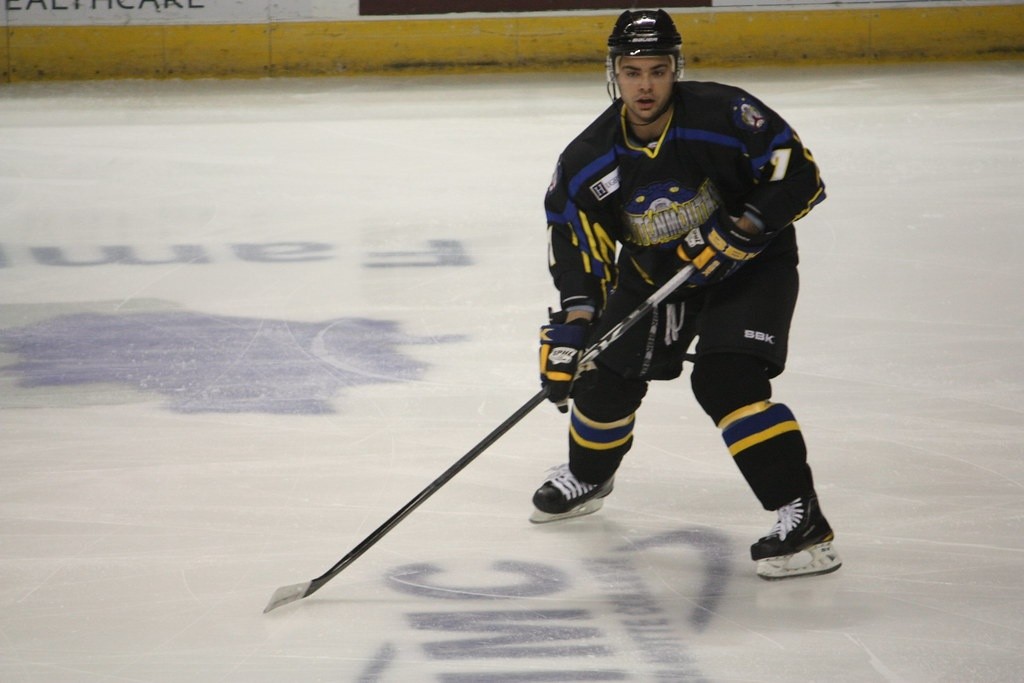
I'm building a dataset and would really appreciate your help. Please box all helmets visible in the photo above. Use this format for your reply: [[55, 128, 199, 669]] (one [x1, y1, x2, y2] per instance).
[[606, 9, 685, 83]]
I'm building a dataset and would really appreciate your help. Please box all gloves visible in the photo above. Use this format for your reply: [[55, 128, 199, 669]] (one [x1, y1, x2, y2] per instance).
[[538, 317, 588, 414], [674, 206, 760, 301]]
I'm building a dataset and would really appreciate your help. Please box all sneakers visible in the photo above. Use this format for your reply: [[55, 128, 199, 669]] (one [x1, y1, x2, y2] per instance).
[[750, 488, 842, 579], [528, 462, 615, 523]]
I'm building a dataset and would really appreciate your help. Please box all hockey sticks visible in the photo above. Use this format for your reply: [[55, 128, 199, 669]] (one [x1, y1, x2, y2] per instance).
[[262, 267, 699, 614]]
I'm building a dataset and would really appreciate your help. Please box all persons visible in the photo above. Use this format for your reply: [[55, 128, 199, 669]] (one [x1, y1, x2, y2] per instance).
[[530, 9, 845, 578]]
